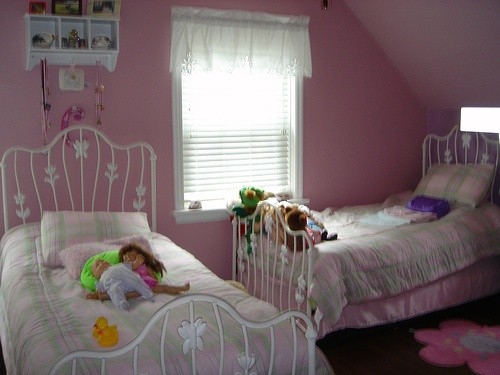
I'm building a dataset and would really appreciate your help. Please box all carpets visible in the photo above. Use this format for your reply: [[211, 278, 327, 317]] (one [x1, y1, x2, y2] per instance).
[[413, 321, 500, 375]]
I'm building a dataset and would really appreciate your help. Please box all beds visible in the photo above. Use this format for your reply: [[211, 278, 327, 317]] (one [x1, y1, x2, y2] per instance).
[[0, 126, 331, 375], [227, 125, 500, 342]]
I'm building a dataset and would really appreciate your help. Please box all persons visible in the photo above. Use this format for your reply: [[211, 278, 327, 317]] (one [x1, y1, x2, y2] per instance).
[[91, 254, 155, 307], [86, 244, 190, 300]]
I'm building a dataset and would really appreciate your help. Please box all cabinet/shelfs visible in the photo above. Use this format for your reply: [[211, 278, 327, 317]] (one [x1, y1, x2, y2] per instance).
[[23, 14, 119, 72]]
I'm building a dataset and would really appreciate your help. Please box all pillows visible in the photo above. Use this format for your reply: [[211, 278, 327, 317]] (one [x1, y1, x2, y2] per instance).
[[386, 163, 495, 223], [41, 210, 163, 292]]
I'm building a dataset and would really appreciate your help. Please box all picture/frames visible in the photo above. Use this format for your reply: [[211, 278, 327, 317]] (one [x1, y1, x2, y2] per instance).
[[51, 0, 82, 17], [28, 0, 47, 16], [87, 0, 121, 19]]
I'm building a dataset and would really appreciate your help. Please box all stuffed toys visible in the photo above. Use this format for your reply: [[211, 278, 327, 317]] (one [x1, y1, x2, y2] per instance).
[[226, 185, 337, 251]]
